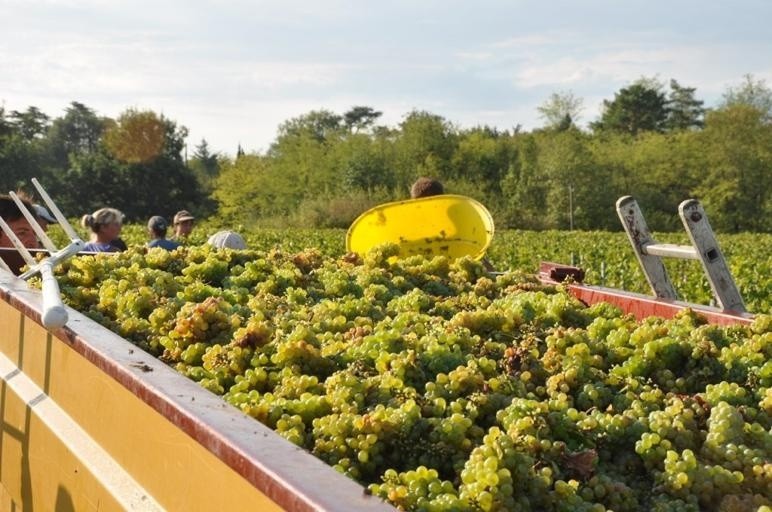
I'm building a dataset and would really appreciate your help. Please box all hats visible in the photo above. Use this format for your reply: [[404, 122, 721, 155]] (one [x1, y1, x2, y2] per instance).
[[32, 203, 58, 225], [172, 210, 195, 225]]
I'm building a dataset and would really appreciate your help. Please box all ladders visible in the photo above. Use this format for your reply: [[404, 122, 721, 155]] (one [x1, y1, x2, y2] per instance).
[[614, 195, 749, 312]]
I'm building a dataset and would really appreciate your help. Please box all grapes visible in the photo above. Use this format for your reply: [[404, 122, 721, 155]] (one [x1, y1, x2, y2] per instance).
[[19, 242, 772, 512]]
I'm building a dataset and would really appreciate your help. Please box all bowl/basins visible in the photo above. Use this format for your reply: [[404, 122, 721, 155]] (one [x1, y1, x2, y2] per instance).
[[347, 194, 494, 266]]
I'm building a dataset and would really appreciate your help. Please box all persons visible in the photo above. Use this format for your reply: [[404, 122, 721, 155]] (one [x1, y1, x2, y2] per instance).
[[145, 217, 179, 251], [0, 194, 38, 277], [30, 204, 58, 232], [410, 178, 444, 198], [81, 208, 124, 253], [169, 210, 195, 243], [203, 230, 247, 251]]
